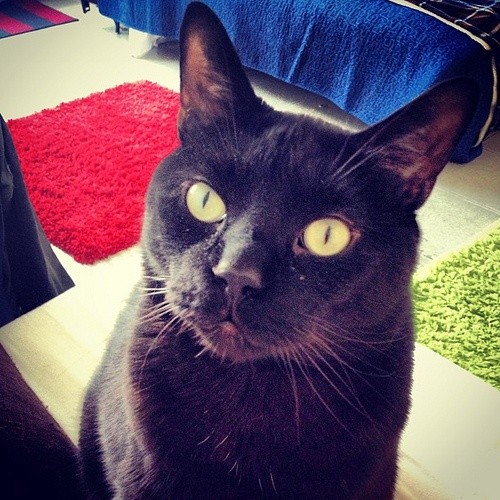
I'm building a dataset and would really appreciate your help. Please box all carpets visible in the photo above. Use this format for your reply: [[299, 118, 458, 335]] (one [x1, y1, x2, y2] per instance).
[[405, 217, 500, 392], [5, 79, 183, 267], [0, 0, 80, 39]]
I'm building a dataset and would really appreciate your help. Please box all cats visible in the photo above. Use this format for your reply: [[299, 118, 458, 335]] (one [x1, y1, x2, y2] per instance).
[[79, 1, 495, 500]]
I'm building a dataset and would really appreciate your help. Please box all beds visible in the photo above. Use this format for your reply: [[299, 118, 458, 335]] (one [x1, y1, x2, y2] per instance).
[[94, 0, 500, 165]]
